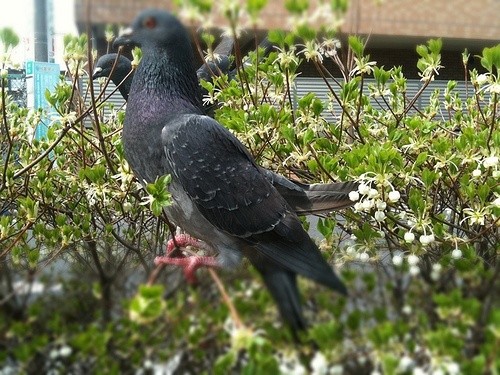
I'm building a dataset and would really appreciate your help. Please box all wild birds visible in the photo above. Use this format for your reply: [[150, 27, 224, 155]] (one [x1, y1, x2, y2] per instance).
[[112, 9, 351, 351], [90, 30, 275, 121]]
